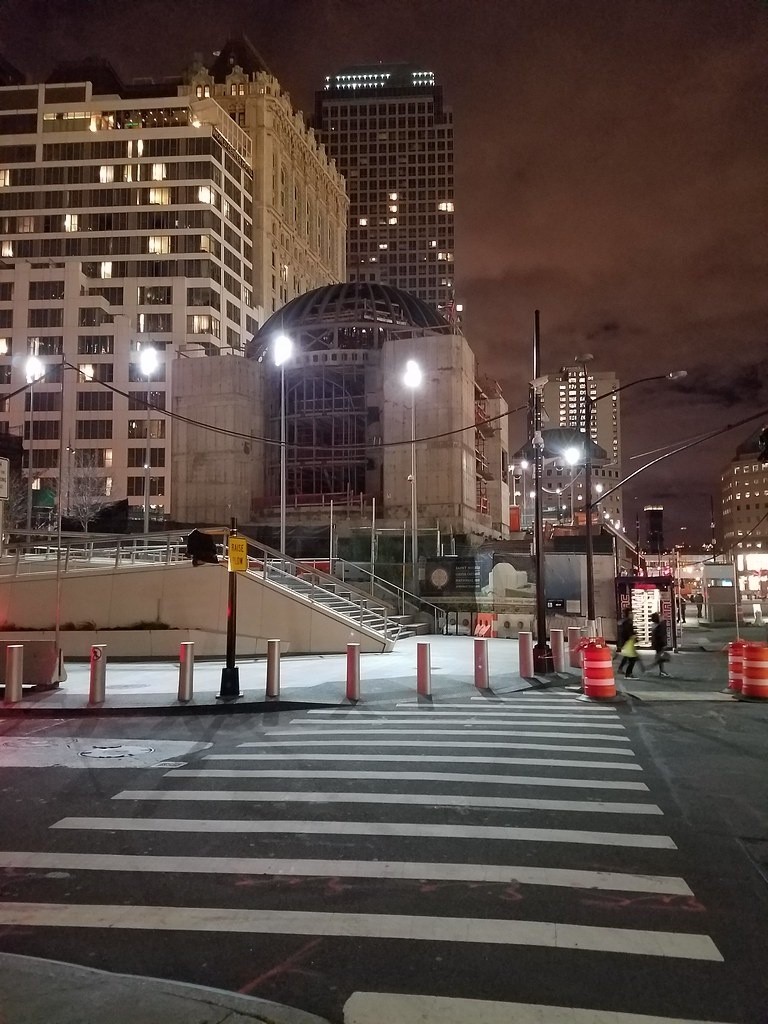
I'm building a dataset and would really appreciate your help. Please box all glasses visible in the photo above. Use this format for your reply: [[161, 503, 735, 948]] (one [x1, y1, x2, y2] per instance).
[[629, 613, 632, 614]]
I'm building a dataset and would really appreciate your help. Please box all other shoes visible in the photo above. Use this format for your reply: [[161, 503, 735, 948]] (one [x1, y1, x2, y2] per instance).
[[677, 620, 679, 623], [617, 670, 626, 674], [659, 671, 670, 678], [682, 621, 686, 623], [624, 675, 640, 680]]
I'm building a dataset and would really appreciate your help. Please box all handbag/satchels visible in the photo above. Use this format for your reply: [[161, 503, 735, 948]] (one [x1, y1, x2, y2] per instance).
[[659, 652, 671, 662]]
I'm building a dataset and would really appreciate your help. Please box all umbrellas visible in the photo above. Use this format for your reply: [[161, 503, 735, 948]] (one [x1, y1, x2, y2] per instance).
[[513, 428, 611, 467]]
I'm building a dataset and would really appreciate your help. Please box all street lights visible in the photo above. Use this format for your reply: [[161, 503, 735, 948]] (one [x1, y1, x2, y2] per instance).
[[140, 349, 155, 543], [274, 337, 293, 568], [24, 360, 40, 553], [403, 369, 422, 602], [586, 370, 688, 638], [534, 443, 582, 675]]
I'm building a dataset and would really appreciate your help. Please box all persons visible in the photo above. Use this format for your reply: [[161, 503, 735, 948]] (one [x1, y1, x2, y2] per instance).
[[621, 634, 637, 680], [676, 597, 687, 623], [646, 613, 670, 677], [617, 608, 644, 676], [696, 593, 703, 618]]
